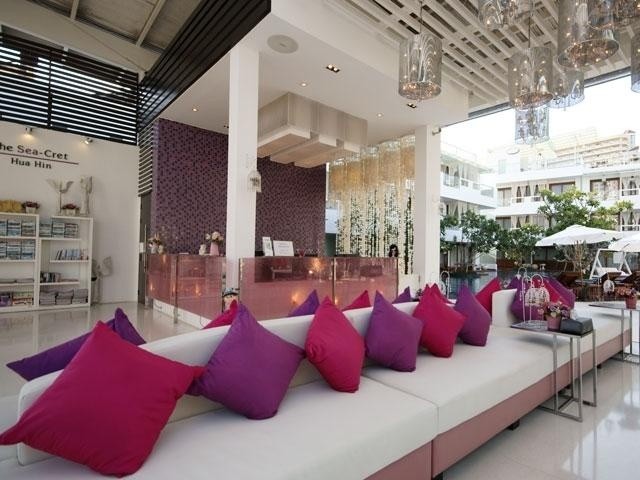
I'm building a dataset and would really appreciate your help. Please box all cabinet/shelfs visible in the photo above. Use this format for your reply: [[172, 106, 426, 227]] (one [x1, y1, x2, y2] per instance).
[[0, 213, 93, 312]]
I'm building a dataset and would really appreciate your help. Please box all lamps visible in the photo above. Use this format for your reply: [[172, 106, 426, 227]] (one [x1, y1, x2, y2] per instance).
[[479, 0, 640, 144], [84, 137, 93, 145], [25, 127, 33, 136], [258, 93, 367, 169], [399, 0, 441, 99]]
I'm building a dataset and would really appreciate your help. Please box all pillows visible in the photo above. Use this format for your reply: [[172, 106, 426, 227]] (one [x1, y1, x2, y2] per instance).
[[6, 305, 149, 381], [286, 288, 321, 318], [452, 279, 491, 348], [1, 318, 212, 480], [303, 294, 369, 395], [341, 289, 373, 310], [411, 283, 468, 358], [362, 288, 425, 373], [418, 282, 454, 304], [200, 298, 239, 328], [182, 300, 308, 423], [391, 285, 413, 303]]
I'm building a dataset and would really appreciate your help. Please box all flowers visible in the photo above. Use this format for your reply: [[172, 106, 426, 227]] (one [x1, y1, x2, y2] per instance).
[[62, 204, 78, 210], [22, 201, 40, 209], [148, 231, 160, 247], [617, 286, 637, 298], [206, 231, 224, 245]]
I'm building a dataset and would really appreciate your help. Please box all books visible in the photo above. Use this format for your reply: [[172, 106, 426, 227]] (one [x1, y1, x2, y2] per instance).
[[0, 218, 88, 306]]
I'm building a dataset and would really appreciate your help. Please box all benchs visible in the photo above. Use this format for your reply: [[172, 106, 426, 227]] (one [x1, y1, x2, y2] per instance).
[[595, 267, 626, 275]]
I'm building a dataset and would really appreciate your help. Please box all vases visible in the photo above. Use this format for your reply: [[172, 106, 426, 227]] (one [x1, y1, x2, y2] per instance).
[[210, 242, 219, 255], [625, 297, 637, 309], [66, 209, 76, 216], [26, 206, 36, 214], [151, 247, 156, 254]]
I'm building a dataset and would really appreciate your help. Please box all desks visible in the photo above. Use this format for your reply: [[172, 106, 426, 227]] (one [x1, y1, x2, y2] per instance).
[[511, 303, 640, 421]]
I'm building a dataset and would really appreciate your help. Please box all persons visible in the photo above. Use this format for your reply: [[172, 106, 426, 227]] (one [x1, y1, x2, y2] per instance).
[[388, 244, 400, 257]]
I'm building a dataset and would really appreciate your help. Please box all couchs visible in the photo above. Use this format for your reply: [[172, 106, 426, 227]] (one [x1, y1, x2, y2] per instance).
[[11, 297, 573, 477], [492, 287, 633, 383]]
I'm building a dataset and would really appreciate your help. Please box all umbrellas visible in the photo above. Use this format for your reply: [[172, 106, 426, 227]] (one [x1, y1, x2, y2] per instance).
[[535, 223, 623, 287], [608, 231, 640, 254]]
